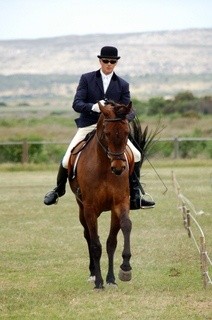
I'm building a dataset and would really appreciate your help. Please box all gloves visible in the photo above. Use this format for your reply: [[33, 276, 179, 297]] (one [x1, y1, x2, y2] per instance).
[[91, 99, 106, 113]]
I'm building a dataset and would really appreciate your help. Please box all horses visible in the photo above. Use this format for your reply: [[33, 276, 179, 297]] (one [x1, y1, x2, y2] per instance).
[[66, 100, 161, 292]]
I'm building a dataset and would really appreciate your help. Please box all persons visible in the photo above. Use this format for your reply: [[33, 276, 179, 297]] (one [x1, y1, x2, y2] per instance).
[[44, 46, 155, 210]]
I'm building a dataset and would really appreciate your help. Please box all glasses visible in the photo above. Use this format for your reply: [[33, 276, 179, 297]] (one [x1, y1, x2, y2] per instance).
[[100, 58, 118, 64]]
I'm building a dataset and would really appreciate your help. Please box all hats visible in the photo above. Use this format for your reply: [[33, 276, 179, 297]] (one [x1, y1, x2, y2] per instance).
[[97, 46, 121, 60]]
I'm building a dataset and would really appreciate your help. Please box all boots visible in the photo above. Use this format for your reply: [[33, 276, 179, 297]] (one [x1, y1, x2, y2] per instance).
[[43, 160, 68, 205], [128, 160, 155, 210]]
